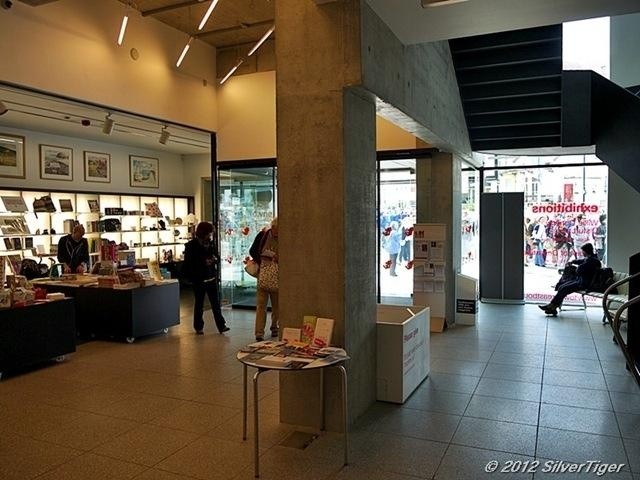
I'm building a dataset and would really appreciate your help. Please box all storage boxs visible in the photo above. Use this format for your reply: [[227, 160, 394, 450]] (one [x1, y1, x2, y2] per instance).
[[377, 304, 430, 405]]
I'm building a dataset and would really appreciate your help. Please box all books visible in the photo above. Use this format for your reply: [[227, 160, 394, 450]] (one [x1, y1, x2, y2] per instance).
[[301, 315, 316, 344], [240, 341, 346, 367], [312, 318, 334, 348], [282, 327, 301, 342]]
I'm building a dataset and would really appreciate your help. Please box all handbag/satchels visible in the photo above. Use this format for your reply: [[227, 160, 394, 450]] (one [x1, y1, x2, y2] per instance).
[[555, 265, 577, 291], [258, 254, 279, 293], [583, 267, 618, 294], [245, 232, 266, 278]]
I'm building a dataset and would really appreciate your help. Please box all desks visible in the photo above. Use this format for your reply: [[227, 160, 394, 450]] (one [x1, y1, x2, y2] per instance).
[[237, 340, 351, 479], [28, 273, 180, 343]]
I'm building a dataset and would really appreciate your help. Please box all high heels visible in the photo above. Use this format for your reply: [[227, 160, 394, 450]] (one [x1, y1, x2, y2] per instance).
[[219, 327, 230, 334], [196, 330, 204, 334]]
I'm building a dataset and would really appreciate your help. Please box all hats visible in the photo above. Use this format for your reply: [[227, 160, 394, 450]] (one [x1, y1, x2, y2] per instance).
[[581, 243, 594, 255]]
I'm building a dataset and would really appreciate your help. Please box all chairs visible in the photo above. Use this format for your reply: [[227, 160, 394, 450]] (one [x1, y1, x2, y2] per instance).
[[558, 261, 630, 323]]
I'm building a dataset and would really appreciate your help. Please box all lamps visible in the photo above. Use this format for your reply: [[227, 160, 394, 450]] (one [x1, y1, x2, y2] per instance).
[[219, 23, 275, 84], [117, 16, 128, 45], [102, 109, 115, 134], [160, 123, 170, 145], [0, 100, 8, 115], [177, 0, 219, 69]]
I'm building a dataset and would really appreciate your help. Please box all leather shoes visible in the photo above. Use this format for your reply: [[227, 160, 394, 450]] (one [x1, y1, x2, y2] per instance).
[[271, 331, 278, 337], [256, 336, 265, 341], [538, 305, 548, 310], [545, 307, 557, 314]]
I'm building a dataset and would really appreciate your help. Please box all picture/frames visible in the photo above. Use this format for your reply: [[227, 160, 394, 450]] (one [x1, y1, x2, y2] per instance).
[[84, 150, 111, 183], [25, 237, 33, 249], [39, 143, 74, 181], [7, 254, 22, 277], [60, 199, 73, 212], [0, 132, 26, 179], [129, 154, 160, 188], [0, 216, 31, 234], [15, 237, 21, 250], [1, 196, 29, 212], [4, 238, 13, 250], [87, 199, 99, 213]]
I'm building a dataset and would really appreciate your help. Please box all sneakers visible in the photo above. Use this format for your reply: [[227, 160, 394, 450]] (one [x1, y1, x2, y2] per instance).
[[542, 265, 546, 267], [535, 263, 540, 266]]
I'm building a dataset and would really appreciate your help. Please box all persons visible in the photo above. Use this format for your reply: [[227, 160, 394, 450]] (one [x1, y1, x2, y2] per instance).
[[185, 222, 230, 334], [554, 221, 574, 275], [524, 218, 530, 267], [531, 217, 550, 267], [593, 215, 607, 261], [570, 213, 593, 259], [57, 224, 90, 275], [386, 221, 400, 277], [527, 224, 535, 258], [399, 218, 410, 264], [249, 219, 279, 341], [565, 214, 574, 226], [539, 244, 601, 314]]
